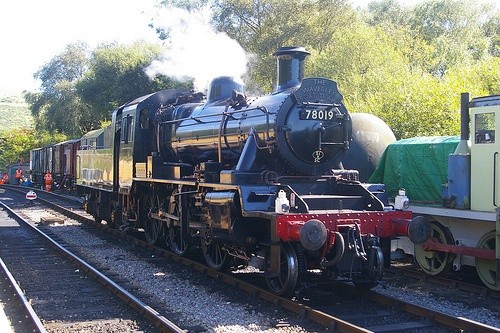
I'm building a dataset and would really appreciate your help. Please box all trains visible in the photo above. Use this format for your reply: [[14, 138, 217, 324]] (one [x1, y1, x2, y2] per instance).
[[25, 44, 435, 300], [342, 91, 500, 295]]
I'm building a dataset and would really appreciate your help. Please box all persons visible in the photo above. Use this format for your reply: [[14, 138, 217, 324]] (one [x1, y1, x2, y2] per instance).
[[15, 167, 22, 185], [44, 171, 52, 191], [1, 172, 8, 184]]
